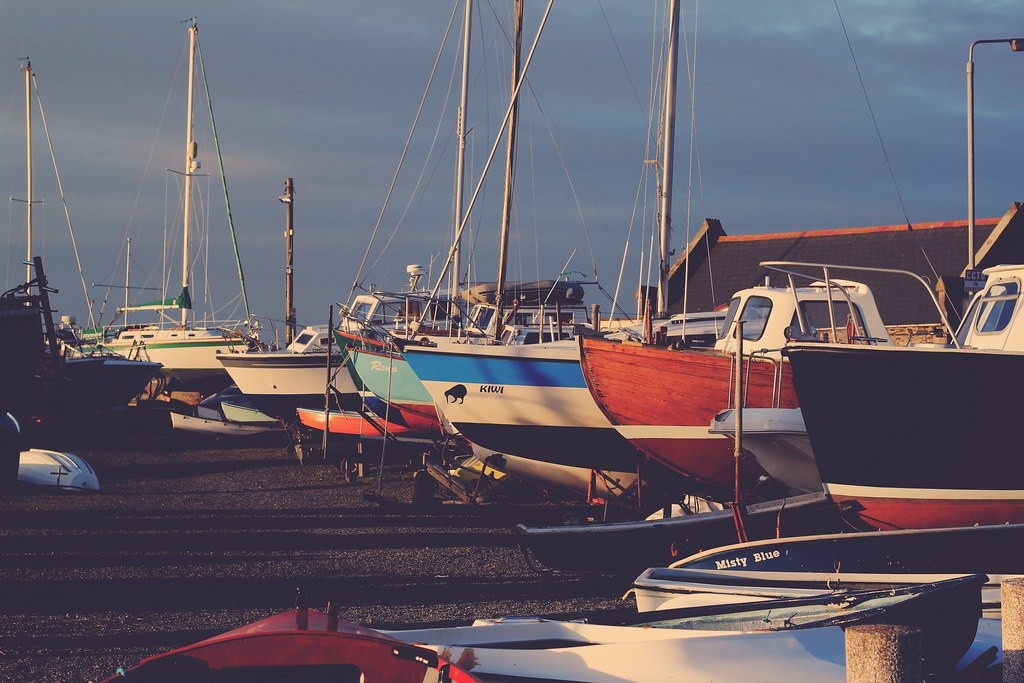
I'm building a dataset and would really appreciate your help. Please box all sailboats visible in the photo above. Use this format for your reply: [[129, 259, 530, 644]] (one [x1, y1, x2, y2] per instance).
[[0, 0, 1024, 683]]
